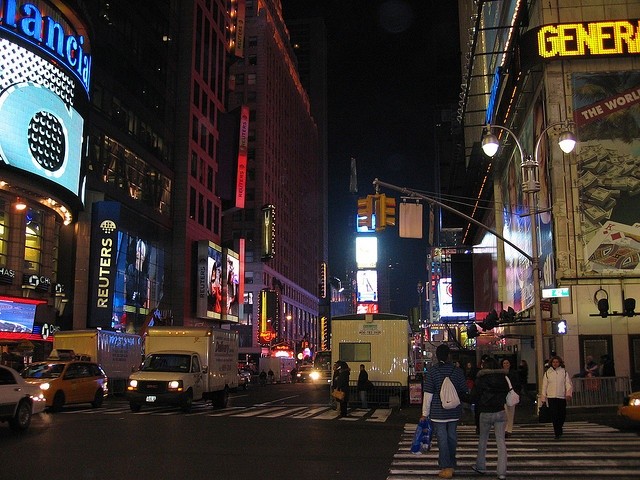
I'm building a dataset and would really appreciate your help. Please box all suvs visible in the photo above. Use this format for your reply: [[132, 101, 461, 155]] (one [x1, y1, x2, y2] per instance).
[[297, 363, 313, 383], [241, 368, 252, 383], [236, 369, 249, 390], [19, 360, 109, 412]]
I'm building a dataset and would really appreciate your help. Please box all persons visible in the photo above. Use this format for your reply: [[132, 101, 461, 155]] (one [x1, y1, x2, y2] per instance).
[[460, 357, 513, 480], [333, 361, 352, 419], [464, 360, 475, 392], [591, 353, 616, 403], [209, 261, 229, 314], [267, 369, 274, 383], [519, 360, 529, 393], [330, 363, 340, 411], [259, 369, 267, 387], [501, 358, 518, 437], [357, 364, 372, 408], [420, 343, 467, 478], [572, 354, 601, 405], [541, 355, 573, 440]]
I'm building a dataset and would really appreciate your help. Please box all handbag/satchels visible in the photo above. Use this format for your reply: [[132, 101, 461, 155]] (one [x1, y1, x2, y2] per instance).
[[539, 404, 552, 423], [410, 419, 433, 454], [503, 373, 521, 410], [332, 387, 345, 399]]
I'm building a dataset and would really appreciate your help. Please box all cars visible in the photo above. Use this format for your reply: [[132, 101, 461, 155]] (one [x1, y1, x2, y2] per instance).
[[414, 357, 433, 380], [0, 365, 46, 431], [618, 392, 639, 422]]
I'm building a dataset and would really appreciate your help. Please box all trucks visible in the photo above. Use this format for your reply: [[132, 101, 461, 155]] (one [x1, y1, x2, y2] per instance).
[[36, 329, 142, 400], [125, 326, 241, 413], [312, 351, 331, 390]]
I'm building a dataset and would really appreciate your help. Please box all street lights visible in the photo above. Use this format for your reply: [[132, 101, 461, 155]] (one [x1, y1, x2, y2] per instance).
[[376, 194, 396, 231], [356, 194, 372, 231], [481, 120, 578, 394], [555, 319, 567, 336]]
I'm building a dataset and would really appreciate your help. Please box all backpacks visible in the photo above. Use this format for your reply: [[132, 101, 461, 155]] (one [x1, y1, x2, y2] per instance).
[[431, 363, 462, 410]]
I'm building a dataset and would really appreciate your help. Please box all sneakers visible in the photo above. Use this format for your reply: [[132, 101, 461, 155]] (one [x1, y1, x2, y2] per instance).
[[497, 474, 506, 480], [471, 464, 486, 474], [439, 468, 454, 478]]
